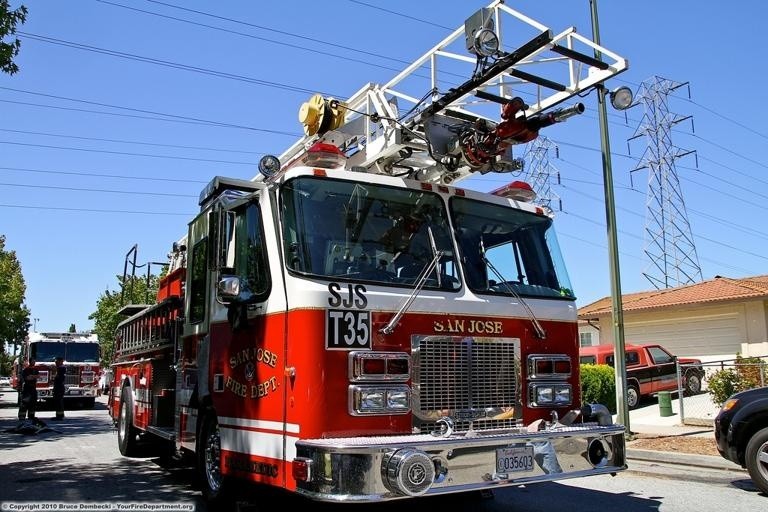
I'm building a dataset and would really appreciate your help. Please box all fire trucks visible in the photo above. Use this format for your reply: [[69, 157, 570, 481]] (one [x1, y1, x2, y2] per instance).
[[13, 334, 100, 399], [108, 1, 634, 504]]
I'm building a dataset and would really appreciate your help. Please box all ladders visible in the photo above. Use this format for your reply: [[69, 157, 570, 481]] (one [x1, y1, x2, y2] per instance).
[[173, 0, 625, 265]]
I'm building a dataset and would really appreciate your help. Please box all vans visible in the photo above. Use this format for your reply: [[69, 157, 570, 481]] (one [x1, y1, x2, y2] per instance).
[[0, 377, 10, 385]]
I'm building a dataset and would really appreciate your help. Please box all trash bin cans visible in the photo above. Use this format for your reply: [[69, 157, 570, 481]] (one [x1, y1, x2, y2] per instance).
[[658, 391, 672, 417]]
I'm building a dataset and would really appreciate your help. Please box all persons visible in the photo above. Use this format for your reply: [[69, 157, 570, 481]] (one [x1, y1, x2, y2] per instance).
[[18, 358, 40, 420], [51, 356, 65, 421]]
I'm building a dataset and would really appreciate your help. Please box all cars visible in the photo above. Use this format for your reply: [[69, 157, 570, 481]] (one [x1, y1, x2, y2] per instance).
[[580, 344, 704, 410], [98, 369, 114, 394], [716, 387, 768, 494]]
